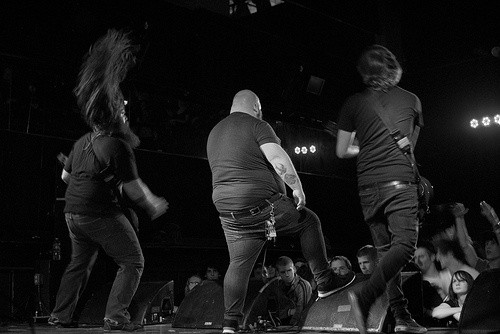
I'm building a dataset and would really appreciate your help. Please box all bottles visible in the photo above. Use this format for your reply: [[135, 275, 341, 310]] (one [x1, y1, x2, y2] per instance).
[[256, 316, 263, 329], [52, 237, 62, 259]]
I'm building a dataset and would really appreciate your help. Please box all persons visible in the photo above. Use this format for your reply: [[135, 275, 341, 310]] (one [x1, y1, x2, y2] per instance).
[[336, 45, 428, 334], [172, 224, 486, 327], [48, 31, 169, 329], [450, 201, 500, 273], [206, 90, 357, 334]]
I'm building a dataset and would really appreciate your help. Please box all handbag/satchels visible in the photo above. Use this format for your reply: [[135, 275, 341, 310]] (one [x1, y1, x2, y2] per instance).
[[418, 176, 435, 230]]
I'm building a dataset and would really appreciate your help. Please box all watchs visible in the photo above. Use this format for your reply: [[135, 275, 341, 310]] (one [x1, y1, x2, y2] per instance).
[[493, 221, 500, 230]]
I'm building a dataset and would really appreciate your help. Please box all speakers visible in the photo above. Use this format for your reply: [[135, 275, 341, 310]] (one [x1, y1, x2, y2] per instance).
[[77, 280, 174, 326], [457, 268, 500, 334], [172, 276, 281, 330], [302, 271, 423, 333], [39, 260, 70, 308]]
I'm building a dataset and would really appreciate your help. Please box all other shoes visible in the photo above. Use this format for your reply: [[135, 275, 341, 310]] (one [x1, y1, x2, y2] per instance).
[[222, 319, 240, 334], [48, 314, 79, 328], [318, 270, 356, 298], [394, 318, 427, 333], [104, 317, 145, 333]]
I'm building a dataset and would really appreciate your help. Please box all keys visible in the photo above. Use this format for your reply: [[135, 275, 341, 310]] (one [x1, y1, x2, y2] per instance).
[[266, 215, 276, 240]]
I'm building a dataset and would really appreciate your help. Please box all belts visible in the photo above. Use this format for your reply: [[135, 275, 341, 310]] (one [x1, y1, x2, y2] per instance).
[[219, 192, 282, 219], [357, 179, 416, 190]]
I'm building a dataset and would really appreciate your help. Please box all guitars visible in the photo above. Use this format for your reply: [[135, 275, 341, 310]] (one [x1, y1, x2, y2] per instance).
[[57, 152, 139, 236], [317, 115, 434, 216]]
